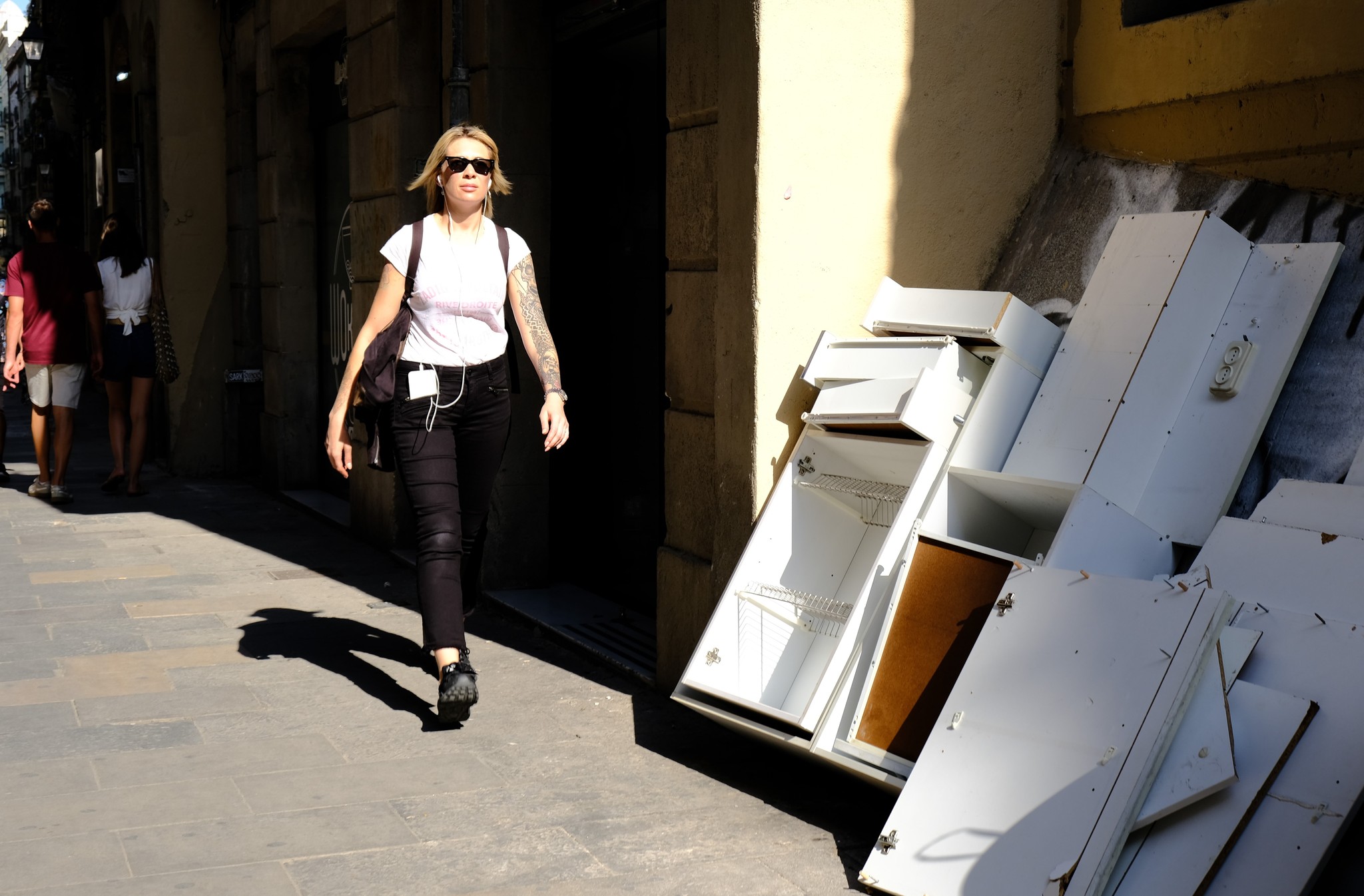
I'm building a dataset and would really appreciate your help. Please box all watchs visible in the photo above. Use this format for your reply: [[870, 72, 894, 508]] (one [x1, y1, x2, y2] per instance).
[[544, 387, 567, 405]]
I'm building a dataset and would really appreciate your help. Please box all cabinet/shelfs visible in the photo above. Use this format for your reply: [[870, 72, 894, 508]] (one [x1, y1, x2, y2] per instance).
[[671, 275, 1066, 751]]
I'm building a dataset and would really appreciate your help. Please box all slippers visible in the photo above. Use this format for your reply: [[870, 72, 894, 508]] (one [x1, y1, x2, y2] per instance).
[[101, 472, 125, 492], [128, 484, 149, 496]]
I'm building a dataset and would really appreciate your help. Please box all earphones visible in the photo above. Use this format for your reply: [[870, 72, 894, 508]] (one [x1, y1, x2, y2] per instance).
[[487, 177, 492, 189], [437, 174, 442, 187]]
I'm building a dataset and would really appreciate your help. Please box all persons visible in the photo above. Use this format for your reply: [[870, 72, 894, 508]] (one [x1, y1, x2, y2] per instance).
[[97, 212, 157, 498], [324, 124, 571, 726], [0, 279, 24, 489], [3, 198, 103, 501]]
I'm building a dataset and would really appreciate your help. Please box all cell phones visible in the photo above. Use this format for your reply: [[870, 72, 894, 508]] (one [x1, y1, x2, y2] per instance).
[[408, 370, 439, 400]]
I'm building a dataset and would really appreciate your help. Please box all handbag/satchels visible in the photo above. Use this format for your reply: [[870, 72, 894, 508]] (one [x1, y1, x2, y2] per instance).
[[148, 307, 180, 383], [363, 401, 397, 473]]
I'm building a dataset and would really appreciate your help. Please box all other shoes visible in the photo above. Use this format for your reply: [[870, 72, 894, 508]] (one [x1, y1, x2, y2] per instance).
[[28, 475, 51, 498], [417, 645, 477, 678], [436, 662, 480, 723], [50, 484, 73, 504], [0, 465, 11, 482]]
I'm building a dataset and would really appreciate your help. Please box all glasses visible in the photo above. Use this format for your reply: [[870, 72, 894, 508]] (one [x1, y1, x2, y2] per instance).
[[438, 155, 495, 174]]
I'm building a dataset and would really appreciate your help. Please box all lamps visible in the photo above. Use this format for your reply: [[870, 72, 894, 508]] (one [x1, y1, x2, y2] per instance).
[[18, 18, 67, 64], [448, 64, 489, 125]]
